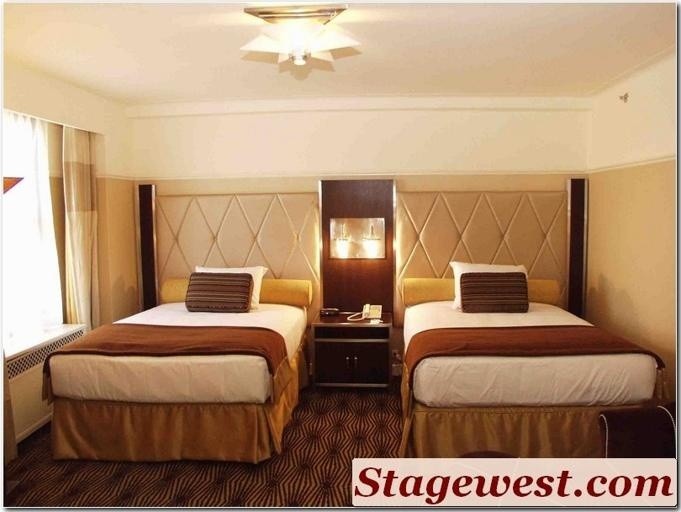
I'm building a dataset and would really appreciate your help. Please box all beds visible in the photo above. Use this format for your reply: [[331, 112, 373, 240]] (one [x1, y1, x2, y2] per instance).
[[400, 299, 664, 458], [41, 305, 310, 464]]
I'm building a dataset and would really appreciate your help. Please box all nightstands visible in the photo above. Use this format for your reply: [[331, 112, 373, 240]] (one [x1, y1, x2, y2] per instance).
[[310, 310, 392, 393]]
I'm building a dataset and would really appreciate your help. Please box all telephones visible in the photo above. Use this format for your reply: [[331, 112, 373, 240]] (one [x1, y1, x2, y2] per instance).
[[362, 304, 382, 320]]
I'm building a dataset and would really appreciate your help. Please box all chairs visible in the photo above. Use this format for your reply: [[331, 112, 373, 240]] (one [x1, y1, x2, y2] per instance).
[[597, 400, 676, 459]]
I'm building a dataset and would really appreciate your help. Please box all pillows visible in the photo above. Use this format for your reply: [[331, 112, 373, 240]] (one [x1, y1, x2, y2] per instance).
[[157, 266, 312, 314], [401, 261, 560, 314]]
